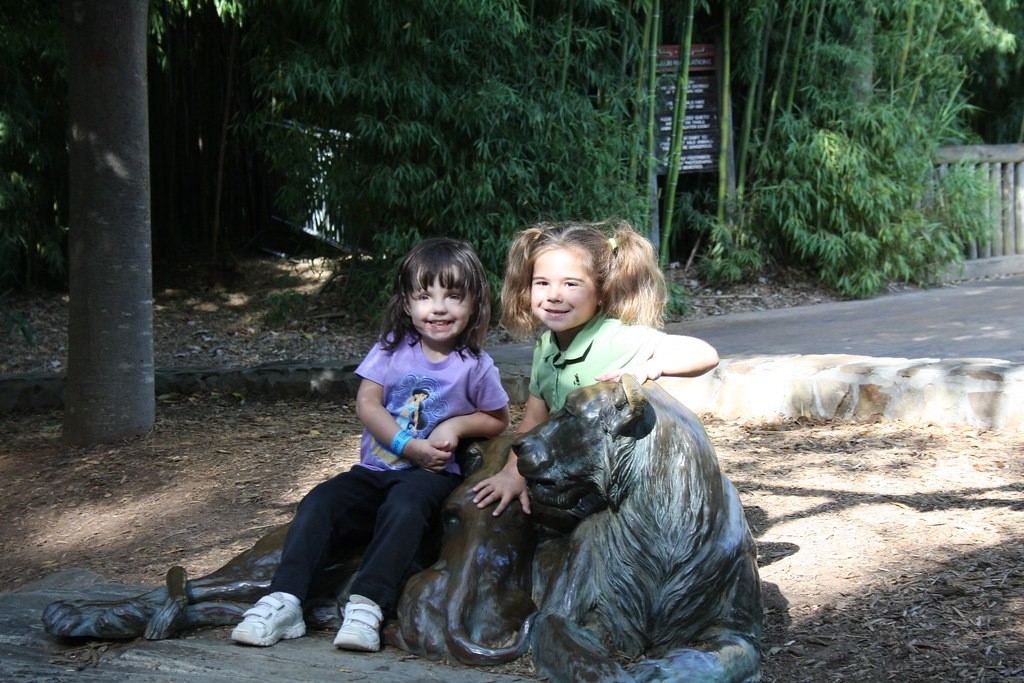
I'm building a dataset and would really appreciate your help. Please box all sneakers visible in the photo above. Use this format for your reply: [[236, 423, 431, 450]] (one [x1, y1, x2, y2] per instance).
[[333, 594, 384, 652], [231, 591, 309, 647]]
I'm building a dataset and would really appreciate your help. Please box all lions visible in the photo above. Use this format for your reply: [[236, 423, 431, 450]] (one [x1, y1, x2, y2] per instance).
[[41, 375, 763, 683]]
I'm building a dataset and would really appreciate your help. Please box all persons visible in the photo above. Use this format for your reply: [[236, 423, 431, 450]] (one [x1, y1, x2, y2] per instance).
[[230, 237, 509, 650], [473, 223, 719, 515]]
